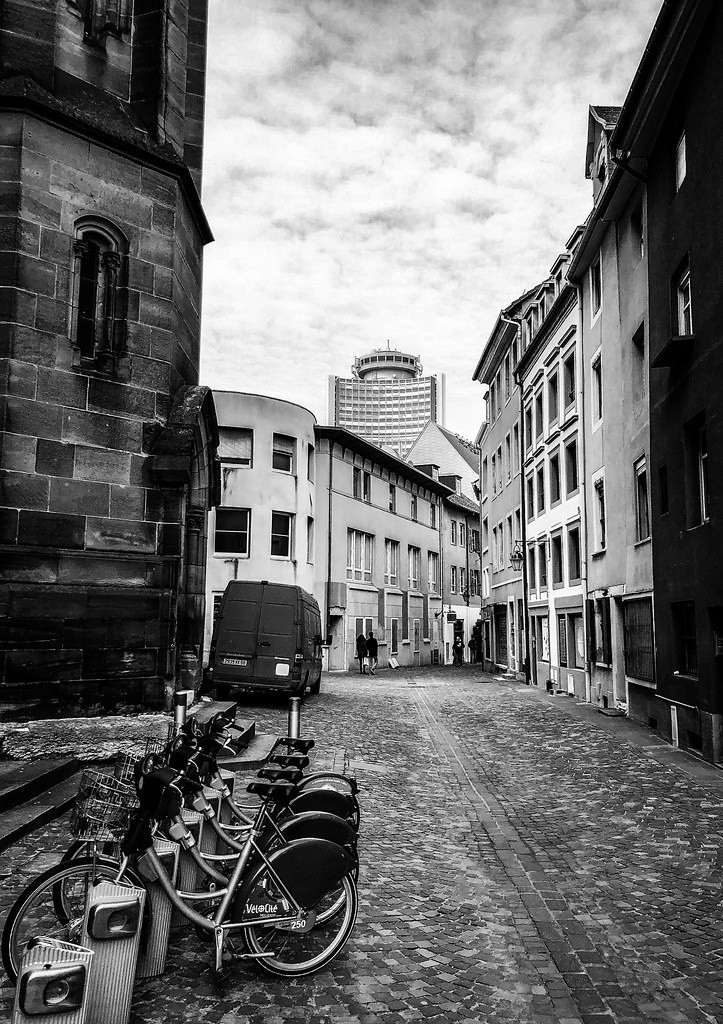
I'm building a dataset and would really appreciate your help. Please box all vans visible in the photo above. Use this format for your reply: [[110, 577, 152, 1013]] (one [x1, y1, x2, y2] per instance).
[[204, 580, 324, 703]]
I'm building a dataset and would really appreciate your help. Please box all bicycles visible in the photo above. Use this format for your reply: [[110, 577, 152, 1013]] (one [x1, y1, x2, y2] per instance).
[[455, 653, 462, 667], [0, 711, 360, 991]]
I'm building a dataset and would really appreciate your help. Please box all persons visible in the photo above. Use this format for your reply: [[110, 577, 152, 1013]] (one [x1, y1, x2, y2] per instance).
[[356, 634, 367, 674], [452, 633, 478, 668], [366, 632, 378, 675]]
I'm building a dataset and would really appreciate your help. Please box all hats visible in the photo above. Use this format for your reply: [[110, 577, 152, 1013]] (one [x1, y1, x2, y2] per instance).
[[456, 636, 462, 642]]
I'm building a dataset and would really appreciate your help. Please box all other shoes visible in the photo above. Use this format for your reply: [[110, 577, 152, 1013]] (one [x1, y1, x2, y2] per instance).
[[360, 672, 362, 674], [362, 670, 365, 673], [371, 670, 375, 674]]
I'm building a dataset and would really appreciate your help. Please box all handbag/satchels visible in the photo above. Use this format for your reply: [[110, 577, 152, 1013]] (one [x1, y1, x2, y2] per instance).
[[363, 657, 369, 666]]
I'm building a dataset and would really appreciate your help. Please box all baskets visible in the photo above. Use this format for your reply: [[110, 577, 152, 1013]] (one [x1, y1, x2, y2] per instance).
[[144, 736, 170, 772], [168, 720, 199, 751], [112, 743, 169, 814], [70, 767, 141, 843]]
